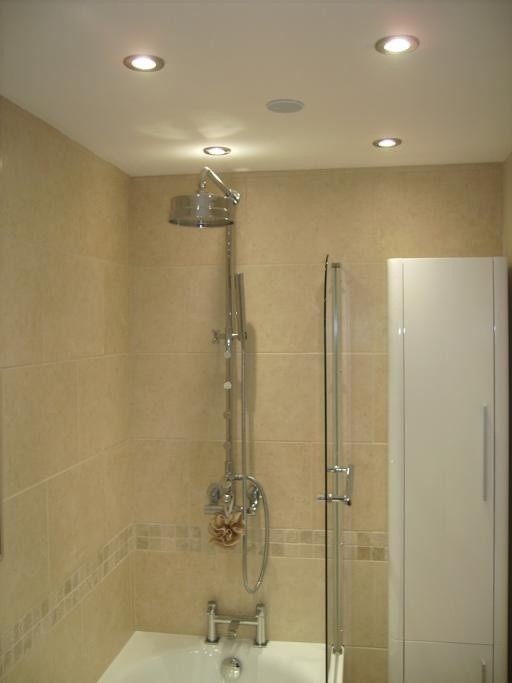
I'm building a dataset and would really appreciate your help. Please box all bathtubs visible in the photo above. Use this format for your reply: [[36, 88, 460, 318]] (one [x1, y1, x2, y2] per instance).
[[96, 629, 345, 683]]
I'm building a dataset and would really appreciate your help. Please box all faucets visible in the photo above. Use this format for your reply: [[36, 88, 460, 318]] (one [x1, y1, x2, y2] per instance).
[[203, 598, 270, 647]]
